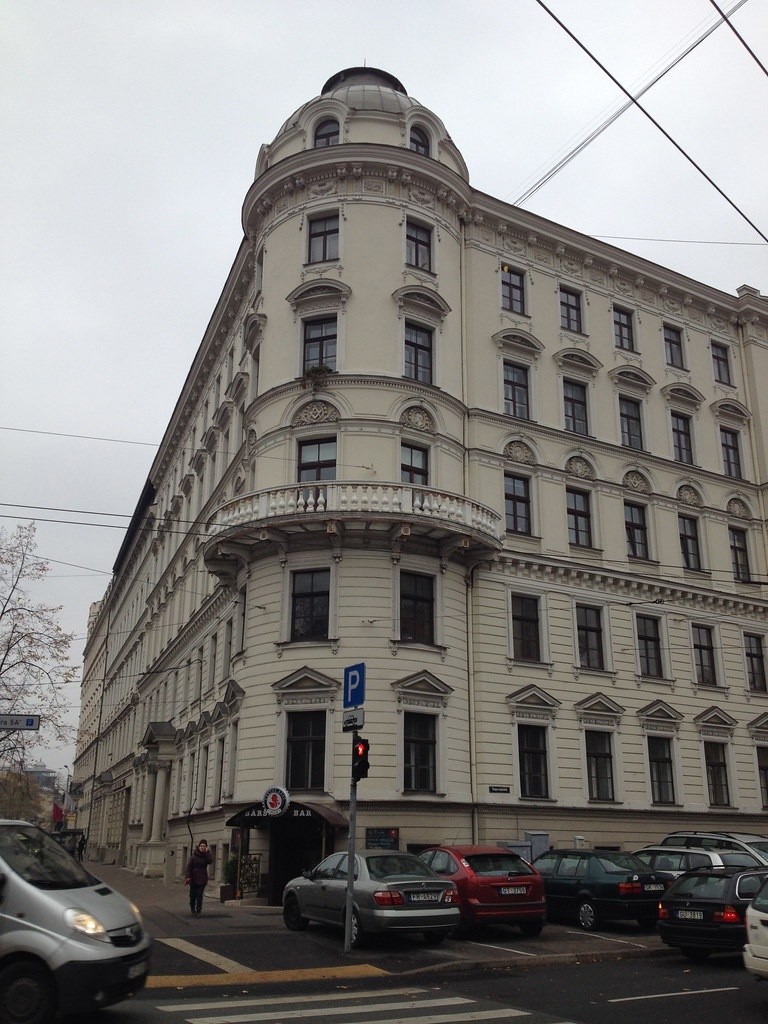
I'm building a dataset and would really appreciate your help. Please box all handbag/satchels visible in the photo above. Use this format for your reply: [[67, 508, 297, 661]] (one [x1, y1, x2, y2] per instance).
[[184, 878, 192, 886]]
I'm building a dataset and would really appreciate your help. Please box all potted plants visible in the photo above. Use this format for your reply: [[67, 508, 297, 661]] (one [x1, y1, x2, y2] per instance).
[[220, 860, 243, 903]]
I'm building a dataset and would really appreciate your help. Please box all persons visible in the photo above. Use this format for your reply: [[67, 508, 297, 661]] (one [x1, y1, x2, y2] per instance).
[[65, 833, 86, 862], [184, 839, 213, 918]]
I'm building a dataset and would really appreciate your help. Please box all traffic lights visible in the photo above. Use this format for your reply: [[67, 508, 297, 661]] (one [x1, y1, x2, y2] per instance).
[[351, 736, 370, 779]]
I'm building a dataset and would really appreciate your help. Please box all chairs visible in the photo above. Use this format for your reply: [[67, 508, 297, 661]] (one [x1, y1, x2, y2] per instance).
[[658, 857, 673, 871]]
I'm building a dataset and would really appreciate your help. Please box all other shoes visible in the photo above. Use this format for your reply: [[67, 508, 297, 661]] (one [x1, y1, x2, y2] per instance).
[[191, 908, 195, 913], [197, 911, 201, 918]]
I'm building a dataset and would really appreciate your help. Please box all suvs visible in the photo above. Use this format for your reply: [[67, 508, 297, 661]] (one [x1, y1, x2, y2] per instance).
[[658, 863, 768, 957], [614, 842, 763, 892], [741, 875, 768, 982], [661, 828, 767, 867]]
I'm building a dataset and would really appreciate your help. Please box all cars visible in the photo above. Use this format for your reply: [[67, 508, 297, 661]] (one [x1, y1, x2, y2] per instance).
[[414, 844, 548, 938], [529, 847, 670, 932], [282, 850, 461, 949]]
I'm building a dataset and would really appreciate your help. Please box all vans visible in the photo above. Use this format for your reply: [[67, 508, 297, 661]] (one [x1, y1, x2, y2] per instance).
[[0, 817, 154, 1024]]
